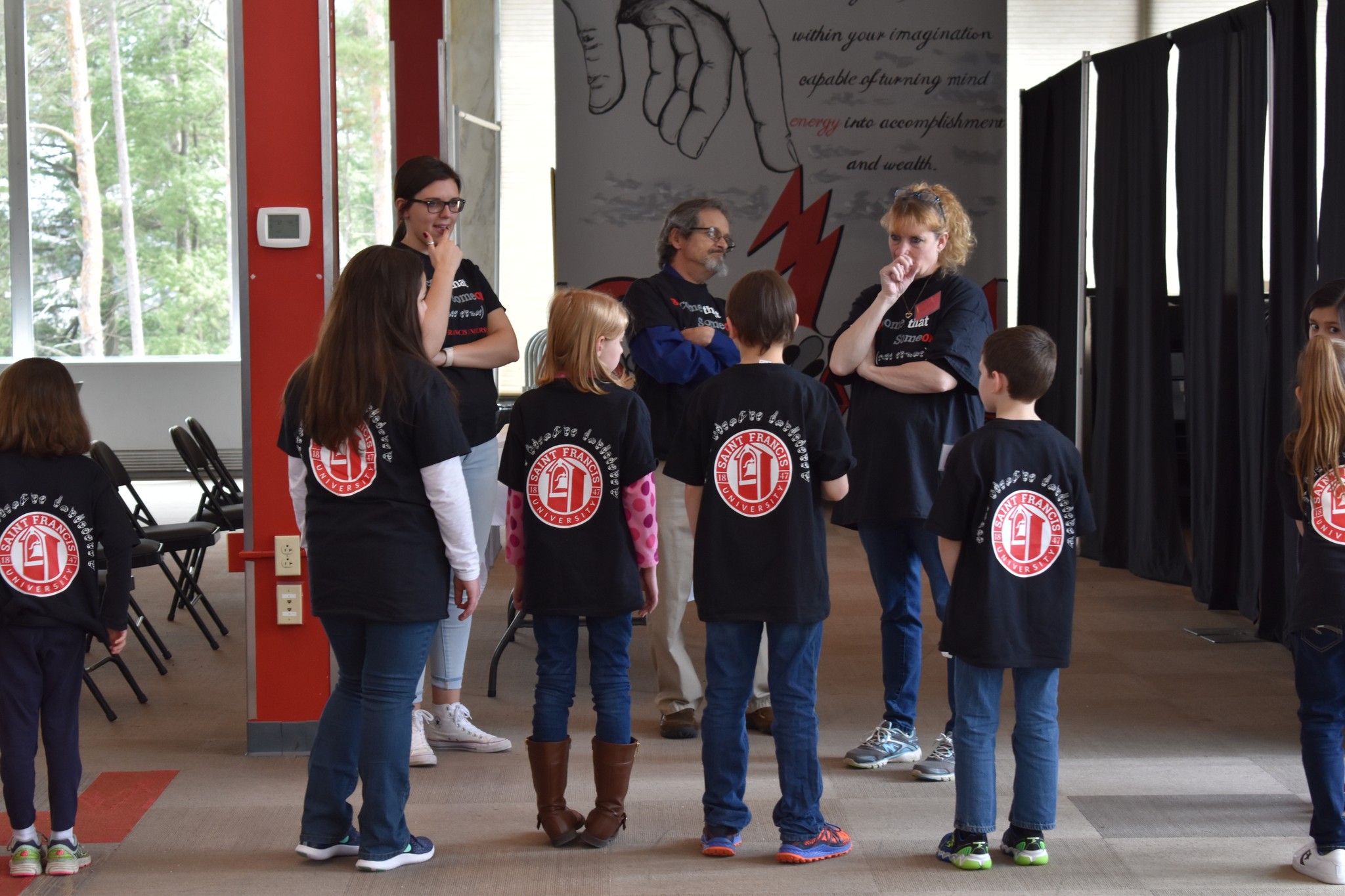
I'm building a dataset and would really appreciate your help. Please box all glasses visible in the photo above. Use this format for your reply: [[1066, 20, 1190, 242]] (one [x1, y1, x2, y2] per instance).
[[894, 188, 946, 222], [687, 226, 735, 252], [404, 196, 466, 214]]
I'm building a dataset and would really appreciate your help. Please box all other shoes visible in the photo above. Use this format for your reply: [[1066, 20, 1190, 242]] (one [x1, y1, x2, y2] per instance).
[[745, 705, 774, 736], [660, 707, 699, 740]]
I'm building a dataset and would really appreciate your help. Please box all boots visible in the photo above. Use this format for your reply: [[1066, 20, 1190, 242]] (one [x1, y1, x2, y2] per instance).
[[581, 735, 640, 848], [525, 734, 586, 847]]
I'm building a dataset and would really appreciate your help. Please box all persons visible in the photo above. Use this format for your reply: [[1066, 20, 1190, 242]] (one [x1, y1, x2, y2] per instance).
[[277, 246, 482, 872], [389, 155, 519, 767], [1274, 331, 1345, 884], [624, 198, 776, 739], [662, 268, 859, 863], [0, 357, 134, 877], [498, 287, 659, 849], [925, 324, 1096, 871], [828, 179, 994, 784], [1307, 279, 1345, 341]]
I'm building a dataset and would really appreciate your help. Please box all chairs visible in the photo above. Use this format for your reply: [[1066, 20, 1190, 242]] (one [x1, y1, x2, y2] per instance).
[[84, 416, 244, 722]]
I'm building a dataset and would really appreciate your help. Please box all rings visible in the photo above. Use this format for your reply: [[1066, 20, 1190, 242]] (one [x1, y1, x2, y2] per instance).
[[426, 242, 434, 245]]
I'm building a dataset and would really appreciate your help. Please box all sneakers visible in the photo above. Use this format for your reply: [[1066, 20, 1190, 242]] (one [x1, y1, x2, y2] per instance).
[[6, 831, 47, 876], [912, 732, 955, 781], [355, 834, 435, 870], [700, 830, 742, 856], [935, 829, 991, 869], [776, 823, 852, 862], [295, 824, 360, 860], [843, 720, 921, 768], [45, 833, 91, 875], [427, 700, 512, 753], [1291, 841, 1345, 884], [408, 709, 437, 765], [1001, 827, 1049, 865]]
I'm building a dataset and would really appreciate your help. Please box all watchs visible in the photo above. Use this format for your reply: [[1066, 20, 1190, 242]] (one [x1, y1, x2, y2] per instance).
[[441, 346, 454, 367]]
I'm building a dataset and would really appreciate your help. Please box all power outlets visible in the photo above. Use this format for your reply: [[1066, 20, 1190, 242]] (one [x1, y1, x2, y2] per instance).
[[274, 536, 301, 576], [276, 585, 303, 625]]
